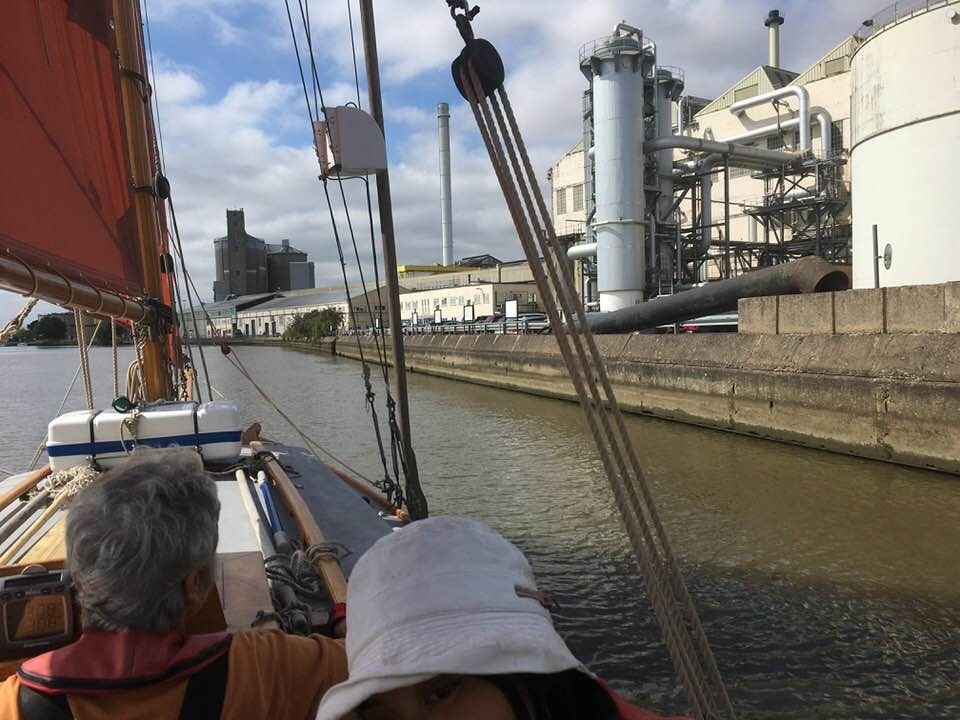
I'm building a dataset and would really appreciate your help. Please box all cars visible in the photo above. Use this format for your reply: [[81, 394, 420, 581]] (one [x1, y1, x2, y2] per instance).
[[402, 313, 550, 333]]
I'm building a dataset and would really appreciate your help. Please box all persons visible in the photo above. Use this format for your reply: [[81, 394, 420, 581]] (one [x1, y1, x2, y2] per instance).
[[316, 515, 693, 720], [0, 445, 349, 720]]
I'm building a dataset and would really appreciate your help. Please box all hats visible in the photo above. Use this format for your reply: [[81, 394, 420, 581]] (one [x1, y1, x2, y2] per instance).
[[314, 513, 600, 720]]
